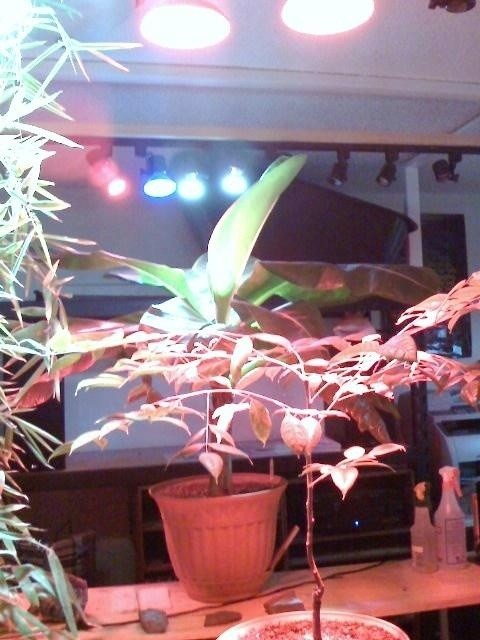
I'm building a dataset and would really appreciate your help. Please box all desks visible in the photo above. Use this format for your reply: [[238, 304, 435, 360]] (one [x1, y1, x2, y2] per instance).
[[0, 557, 480, 640]]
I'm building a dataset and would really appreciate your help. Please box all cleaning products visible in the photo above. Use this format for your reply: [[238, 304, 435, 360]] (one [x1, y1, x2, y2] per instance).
[[432, 465, 468, 570], [409, 479, 439, 575]]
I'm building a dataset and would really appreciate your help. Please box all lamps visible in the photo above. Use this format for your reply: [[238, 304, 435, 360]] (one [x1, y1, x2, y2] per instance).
[[85, 137, 463, 198]]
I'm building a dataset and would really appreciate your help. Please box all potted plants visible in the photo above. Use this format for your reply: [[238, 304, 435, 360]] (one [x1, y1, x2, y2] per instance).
[[5, 151, 316, 605], [43, 266, 480, 640]]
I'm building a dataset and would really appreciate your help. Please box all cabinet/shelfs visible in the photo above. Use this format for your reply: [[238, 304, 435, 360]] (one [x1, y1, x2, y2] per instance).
[[127, 467, 420, 582]]
